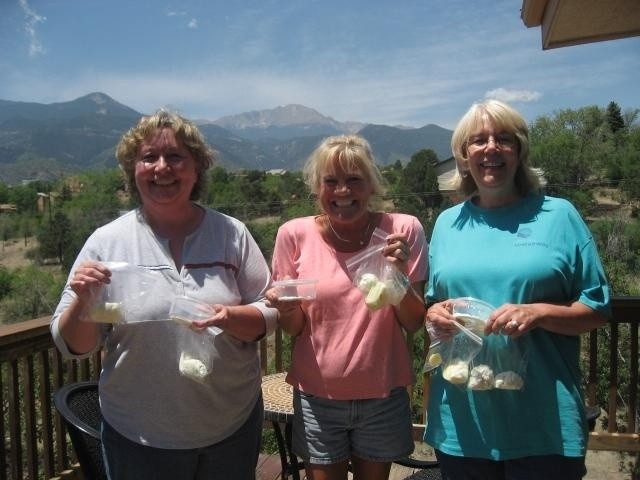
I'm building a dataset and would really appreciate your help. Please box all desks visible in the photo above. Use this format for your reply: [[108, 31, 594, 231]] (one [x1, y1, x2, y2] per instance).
[[261, 371, 353, 480]]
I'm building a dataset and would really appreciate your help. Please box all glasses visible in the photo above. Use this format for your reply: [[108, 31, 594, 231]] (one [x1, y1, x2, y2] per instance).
[[469, 132, 519, 150]]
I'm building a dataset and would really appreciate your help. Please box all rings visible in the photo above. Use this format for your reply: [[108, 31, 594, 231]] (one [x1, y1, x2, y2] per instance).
[[511, 320, 519, 328]]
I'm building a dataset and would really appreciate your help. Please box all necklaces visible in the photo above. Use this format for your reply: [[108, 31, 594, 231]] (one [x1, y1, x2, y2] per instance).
[[326, 213, 374, 245]]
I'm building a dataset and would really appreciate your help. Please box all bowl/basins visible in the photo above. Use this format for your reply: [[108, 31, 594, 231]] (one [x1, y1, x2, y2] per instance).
[[169, 295, 217, 328], [449, 297, 496, 332], [274, 279, 317, 303]]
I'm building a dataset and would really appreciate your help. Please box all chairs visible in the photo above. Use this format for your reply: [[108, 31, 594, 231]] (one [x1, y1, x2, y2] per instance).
[[53, 380, 102, 480], [394, 405, 601, 480]]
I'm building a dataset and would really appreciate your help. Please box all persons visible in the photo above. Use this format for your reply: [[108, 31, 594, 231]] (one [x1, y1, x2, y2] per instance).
[[262, 135, 429, 480], [49, 105, 281, 480], [423, 100, 611, 477]]
